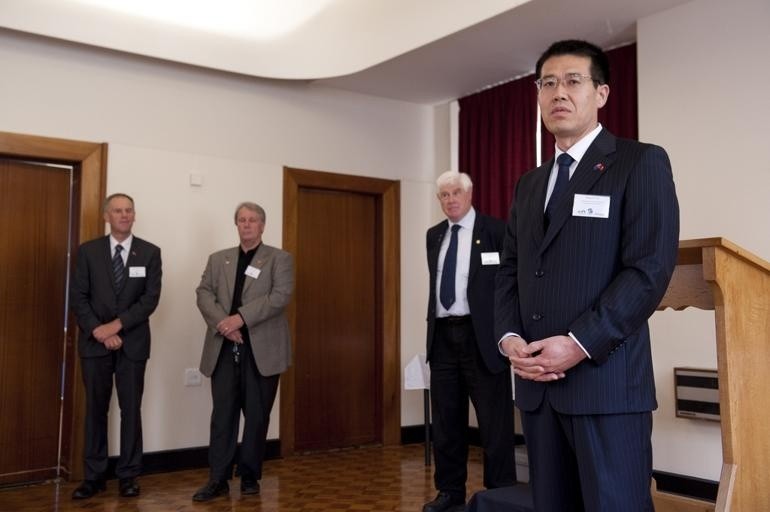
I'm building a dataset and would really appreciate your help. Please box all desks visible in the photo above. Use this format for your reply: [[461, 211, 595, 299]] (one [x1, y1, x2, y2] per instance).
[[402, 351, 521, 467]]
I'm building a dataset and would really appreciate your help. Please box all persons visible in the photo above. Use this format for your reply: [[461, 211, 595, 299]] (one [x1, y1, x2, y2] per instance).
[[426, 172, 508, 512], [192, 200, 295, 502], [493, 37, 680, 512], [68, 193, 163, 500]]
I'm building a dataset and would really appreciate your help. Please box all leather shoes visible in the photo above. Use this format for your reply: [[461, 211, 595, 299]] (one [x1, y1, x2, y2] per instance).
[[423, 489, 466, 512], [72, 480, 106, 499], [119, 478, 140, 497], [240, 474, 260, 493]]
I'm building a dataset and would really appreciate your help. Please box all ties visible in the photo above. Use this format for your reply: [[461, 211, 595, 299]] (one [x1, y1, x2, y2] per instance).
[[440, 223, 462, 311], [112, 245, 125, 289], [193, 477, 230, 501], [544, 152, 574, 217]]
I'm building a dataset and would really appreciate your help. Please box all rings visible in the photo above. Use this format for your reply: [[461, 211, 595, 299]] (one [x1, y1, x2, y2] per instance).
[[225, 328, 231, 331]]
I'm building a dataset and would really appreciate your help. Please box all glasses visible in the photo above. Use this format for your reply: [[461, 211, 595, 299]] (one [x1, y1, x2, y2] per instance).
[[535, 73, 593, 89]]
